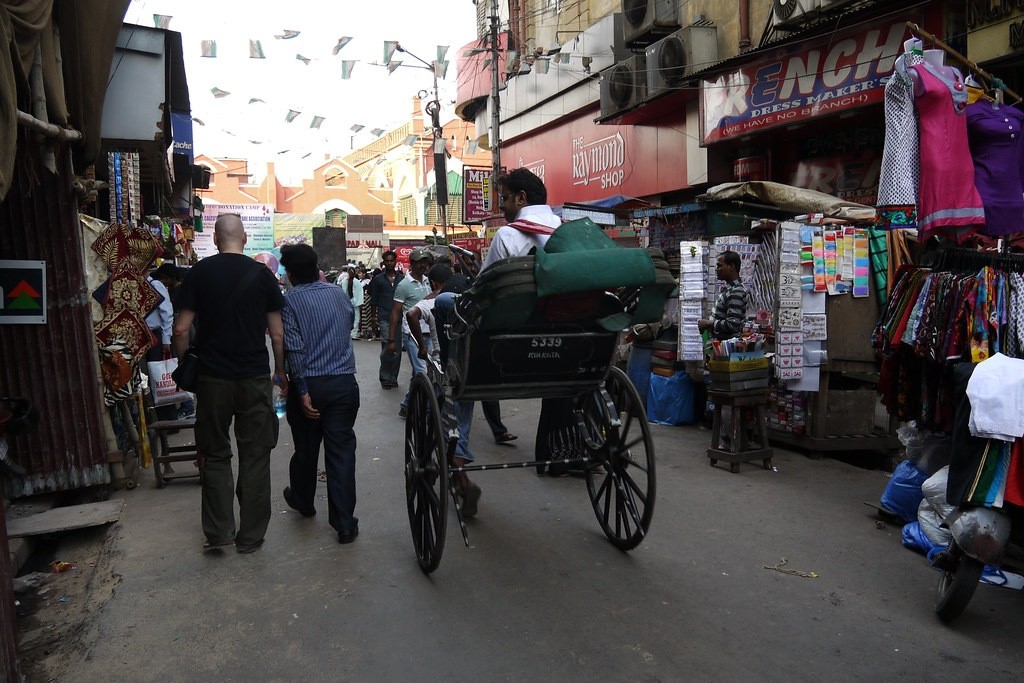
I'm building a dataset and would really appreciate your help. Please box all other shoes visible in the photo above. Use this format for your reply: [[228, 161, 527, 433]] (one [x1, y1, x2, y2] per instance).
[[353, 333, 381, 342], [458, 484, 480, 518], [495, 433, 518, 444]]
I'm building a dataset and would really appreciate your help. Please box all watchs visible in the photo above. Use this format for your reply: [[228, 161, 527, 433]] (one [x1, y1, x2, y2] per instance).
[[387, 338, 395, 344]]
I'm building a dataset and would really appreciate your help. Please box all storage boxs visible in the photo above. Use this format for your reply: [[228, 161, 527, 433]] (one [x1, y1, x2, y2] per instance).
[[707, 360, 769, 391]]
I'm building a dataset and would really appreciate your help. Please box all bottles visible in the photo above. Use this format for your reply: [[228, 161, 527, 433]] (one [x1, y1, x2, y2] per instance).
[[272, 378, 287, 419]]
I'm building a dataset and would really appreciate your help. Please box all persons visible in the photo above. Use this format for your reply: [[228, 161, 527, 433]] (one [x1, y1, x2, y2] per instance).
[[277, 242, 361, 544], [960, 94, 1023, 238], [696, 250, 748, 450], [905, 48, 986, 241], [176, 213, 287, 555], [875, 38, 920, 213], [313, 256, 383, 341], [142, 271, 174, 362], [433, 168, 561, 353], [366, 250, 404, 389], [385, 248, 519, 518]]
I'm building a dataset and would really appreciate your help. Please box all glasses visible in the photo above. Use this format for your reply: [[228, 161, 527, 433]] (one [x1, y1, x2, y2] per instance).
[[499, 191, 513, 205]]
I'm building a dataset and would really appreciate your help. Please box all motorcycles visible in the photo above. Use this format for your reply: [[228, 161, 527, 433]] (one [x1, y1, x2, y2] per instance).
[[929, 352, 1024, 628]]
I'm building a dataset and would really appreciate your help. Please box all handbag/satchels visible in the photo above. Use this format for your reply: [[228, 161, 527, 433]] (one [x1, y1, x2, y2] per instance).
[[148, 351, 194, 408]]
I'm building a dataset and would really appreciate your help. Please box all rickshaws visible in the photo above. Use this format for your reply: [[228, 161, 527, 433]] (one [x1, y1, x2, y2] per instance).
[[403, 216, 679, 573]]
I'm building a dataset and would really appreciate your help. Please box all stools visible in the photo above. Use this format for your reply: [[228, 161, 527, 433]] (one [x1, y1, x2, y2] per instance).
[[707, 388, 774, 473], [150, 418, 201, 489]]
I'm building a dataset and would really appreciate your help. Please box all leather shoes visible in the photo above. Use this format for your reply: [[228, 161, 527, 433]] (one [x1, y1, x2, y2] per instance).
[[283, 487, 316, 517], [339, 526, 359, 543]]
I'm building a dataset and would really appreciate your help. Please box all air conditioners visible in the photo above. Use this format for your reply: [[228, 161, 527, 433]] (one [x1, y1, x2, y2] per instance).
[[645, 25, 718, 97], [621, 0, 682, 50], [599, 54, 646, 117], [772, 0, 854, 28]]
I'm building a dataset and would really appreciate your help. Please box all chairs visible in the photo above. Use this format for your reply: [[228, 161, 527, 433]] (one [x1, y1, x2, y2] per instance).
[[431, 256, 628, 400]]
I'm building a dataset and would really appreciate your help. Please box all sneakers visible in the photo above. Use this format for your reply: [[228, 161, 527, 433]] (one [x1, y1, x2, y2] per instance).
[[203, 540, 235, 549]]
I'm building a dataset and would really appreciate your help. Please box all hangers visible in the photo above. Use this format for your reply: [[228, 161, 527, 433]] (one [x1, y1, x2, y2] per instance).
[[899, 246, 1024, 286]]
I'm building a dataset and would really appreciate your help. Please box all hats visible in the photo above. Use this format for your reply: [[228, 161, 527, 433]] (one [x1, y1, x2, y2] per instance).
[[409, 248, 431, 263], [280, 244, 318, 271]]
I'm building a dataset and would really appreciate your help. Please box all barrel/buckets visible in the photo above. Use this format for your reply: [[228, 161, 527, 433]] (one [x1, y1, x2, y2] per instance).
[[626, 339, 656, 417]]
